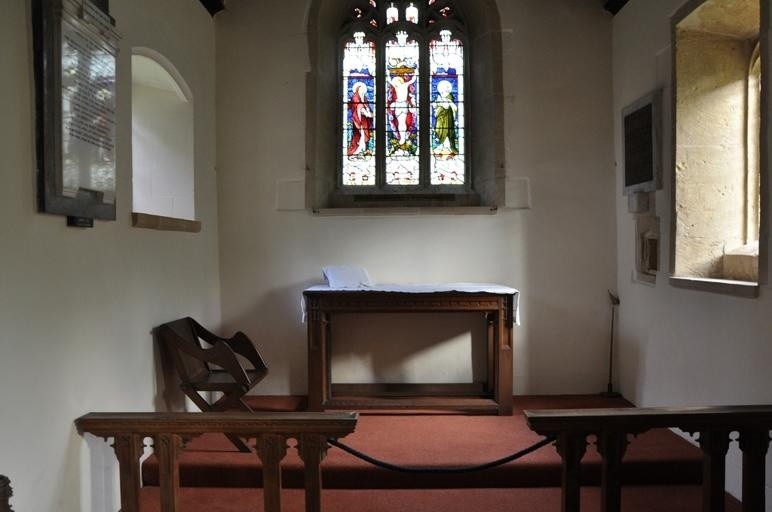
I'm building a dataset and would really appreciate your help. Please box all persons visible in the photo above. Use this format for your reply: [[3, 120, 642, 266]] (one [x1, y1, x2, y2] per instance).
[[387, 69, 419, 145], [431, 79, 458, 159], [346, 82, 373, 157]]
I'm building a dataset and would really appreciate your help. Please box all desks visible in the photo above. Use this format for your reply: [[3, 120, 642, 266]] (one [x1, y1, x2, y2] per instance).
[[303, 284, 516, 415]]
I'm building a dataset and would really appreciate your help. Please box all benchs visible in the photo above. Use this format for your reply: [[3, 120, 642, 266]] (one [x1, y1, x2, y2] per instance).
[[522, 405, 772, 510], [152, 317, 269, 453], [74, 412, 357, 512]]
